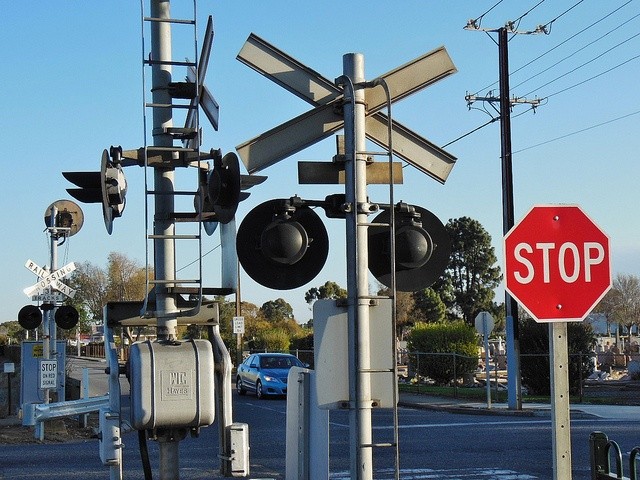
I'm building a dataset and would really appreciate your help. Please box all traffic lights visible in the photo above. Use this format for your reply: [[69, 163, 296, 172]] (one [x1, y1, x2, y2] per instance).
[[209, 152, 268, 224], [62, 149, 127, 234]]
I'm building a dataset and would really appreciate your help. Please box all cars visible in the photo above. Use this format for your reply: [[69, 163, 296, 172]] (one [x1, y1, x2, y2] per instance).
[[236, 353, 306, 399]]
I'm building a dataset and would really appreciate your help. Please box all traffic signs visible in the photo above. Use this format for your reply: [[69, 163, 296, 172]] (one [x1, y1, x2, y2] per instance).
[[24, 259, 76, 301]]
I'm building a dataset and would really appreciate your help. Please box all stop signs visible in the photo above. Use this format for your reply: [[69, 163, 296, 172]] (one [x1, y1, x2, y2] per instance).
[[503, 205, 611, 323]]
